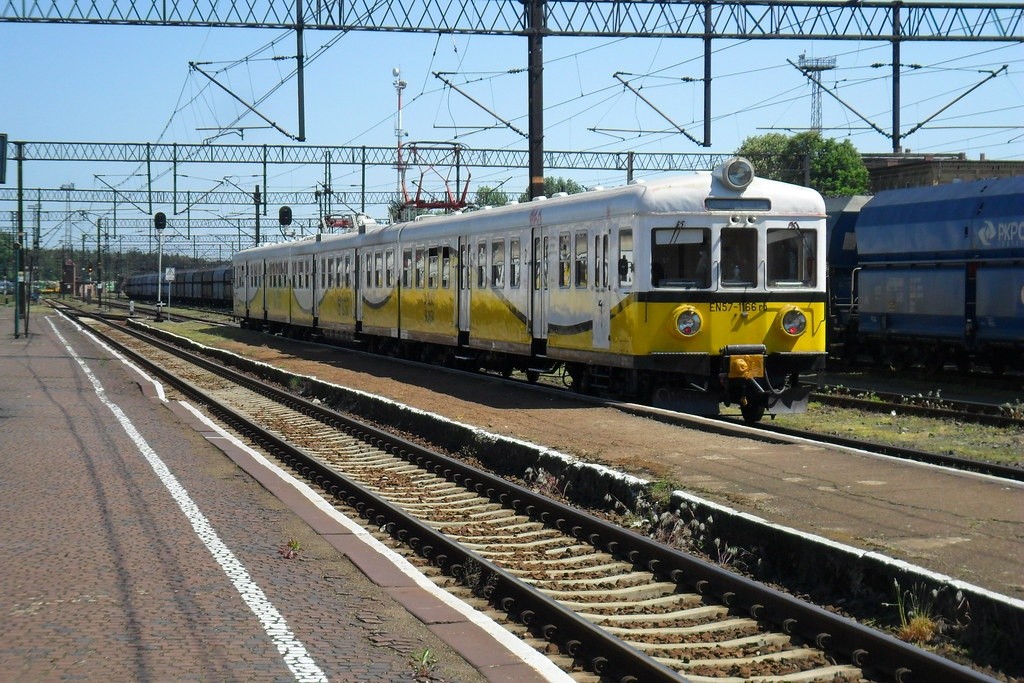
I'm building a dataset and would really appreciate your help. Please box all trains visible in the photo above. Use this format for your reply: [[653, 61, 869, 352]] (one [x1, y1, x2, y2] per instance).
[[124, 175, 1024, 374], [231, 155, 827, 423]]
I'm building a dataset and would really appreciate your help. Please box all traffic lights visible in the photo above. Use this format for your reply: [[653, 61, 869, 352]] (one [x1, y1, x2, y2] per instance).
[[155, 212, 166, 229], [88, 262, 93, 272], [279, 206, 292, 226]]
